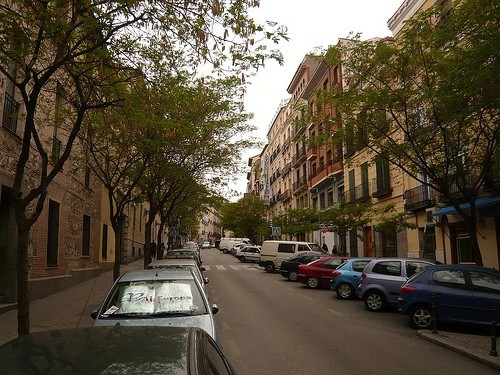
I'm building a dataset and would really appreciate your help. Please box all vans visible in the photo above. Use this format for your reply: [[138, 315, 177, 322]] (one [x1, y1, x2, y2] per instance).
[[215, 240, 221, 248], [219, 238, 251, 254], [259, 240, 326, 274]]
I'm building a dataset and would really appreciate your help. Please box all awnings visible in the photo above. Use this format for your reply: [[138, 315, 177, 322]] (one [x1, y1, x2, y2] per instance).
[[432, 196, 500, 216]]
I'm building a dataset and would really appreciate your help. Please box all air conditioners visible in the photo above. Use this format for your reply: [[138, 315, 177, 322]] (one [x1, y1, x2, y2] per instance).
[[425, 207, 442, 225]]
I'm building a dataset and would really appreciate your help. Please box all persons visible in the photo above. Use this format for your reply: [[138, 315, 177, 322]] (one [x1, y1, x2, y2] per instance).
[[322, 243, 338, 255], [151, 239, 165, 260]]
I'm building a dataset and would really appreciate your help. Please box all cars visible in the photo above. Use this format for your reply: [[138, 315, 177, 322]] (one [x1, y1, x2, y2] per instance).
[[359, 257, 481, 313], [398, 264, 500, 335], [202, 241, 211, 249], [330, 257, 377, 299], [164, 241, 203, 266], [231, 243, 262, 263], [279, 252, 330, 282], [298, 256, 357, 289], [144, 258, 210, 299], [89, 266, 220, 343], [0, 323, 236, 375]]
